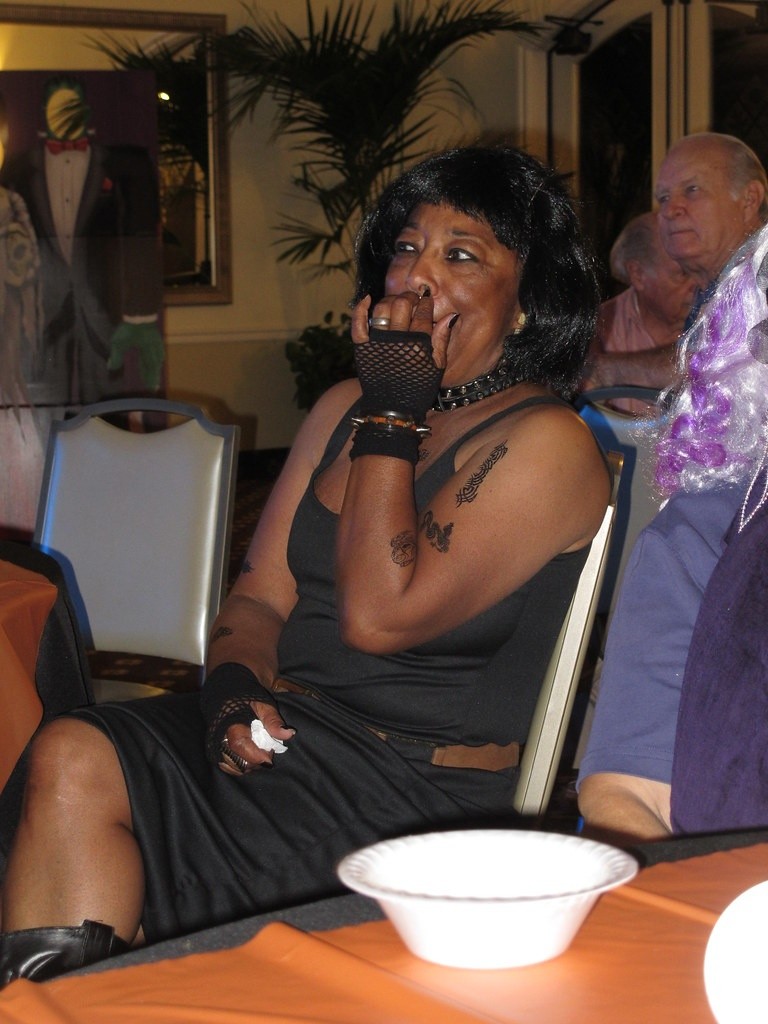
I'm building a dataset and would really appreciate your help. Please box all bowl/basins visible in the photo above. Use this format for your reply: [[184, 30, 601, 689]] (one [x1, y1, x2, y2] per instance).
[[336, 827, 638, 970]]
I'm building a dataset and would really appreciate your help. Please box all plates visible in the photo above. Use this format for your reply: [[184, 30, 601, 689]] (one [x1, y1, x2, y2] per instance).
[[702, 878, 768, 1024]]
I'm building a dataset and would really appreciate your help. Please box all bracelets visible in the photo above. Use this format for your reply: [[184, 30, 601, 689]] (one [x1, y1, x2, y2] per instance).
[[376, 410, 414, 419], [349, 432, 419, 465], [350, 415, 432, 438]]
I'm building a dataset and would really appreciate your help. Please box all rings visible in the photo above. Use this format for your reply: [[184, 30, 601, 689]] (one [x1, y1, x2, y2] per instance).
[[220, 740, 248, 773], [369, 319, 390, 327]]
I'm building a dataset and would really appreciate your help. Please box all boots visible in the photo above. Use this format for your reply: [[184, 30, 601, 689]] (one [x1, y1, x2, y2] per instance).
[[0, 919, 130, 991]]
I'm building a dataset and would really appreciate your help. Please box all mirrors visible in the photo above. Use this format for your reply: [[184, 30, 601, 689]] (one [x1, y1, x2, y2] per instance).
[[0, 1, 232, 308]]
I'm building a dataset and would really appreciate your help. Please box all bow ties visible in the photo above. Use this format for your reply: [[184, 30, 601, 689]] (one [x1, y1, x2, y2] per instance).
[[48, 137, 88, 155]]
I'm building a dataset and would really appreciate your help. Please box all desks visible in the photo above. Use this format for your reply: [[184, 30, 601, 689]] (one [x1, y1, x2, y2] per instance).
[[0, 828, 768, 1023]]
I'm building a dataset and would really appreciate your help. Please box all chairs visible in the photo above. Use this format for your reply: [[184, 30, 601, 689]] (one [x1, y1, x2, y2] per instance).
[[510, 383, 692, 824], [29, 397, 241, 705]]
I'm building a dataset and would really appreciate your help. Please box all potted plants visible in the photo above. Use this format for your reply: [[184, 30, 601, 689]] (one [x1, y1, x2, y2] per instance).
[[46, 1, 554, 410]]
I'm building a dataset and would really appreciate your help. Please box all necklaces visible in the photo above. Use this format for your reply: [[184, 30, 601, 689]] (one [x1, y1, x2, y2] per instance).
[[433, 364, 532, 410]]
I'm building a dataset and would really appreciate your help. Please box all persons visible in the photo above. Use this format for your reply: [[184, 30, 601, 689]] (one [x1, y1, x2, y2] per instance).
[[0, 142, 613, 990], [585, 134, 768, 843]]
